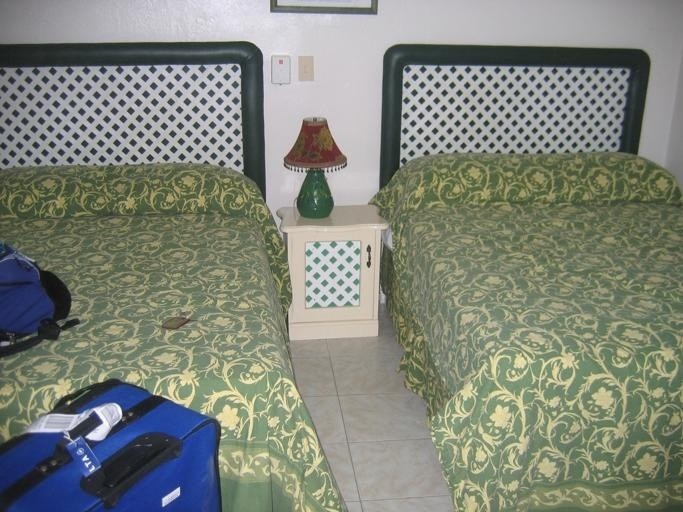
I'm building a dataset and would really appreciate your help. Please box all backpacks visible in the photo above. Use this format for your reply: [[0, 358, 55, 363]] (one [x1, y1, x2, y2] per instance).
[[0, 244, 80, 356]]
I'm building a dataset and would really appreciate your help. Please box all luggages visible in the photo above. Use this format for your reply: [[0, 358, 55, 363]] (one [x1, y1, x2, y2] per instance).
[[0, 379, 221, 512]]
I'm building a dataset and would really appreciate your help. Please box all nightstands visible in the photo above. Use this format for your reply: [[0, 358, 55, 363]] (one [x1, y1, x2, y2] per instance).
[[274, 204, 389, 343]]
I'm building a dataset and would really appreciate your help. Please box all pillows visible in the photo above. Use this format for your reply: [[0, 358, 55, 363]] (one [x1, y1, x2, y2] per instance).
[[0, 161, 260, 221], [386, 151, 677, 209]]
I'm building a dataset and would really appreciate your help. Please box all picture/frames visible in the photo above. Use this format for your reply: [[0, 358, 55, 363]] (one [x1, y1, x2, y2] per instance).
[[270, 0, 379, 16]]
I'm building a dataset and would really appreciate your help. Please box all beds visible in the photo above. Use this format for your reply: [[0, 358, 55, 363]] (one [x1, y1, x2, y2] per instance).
[[380, 43, 683, 511], [0, 40, 305, 512]]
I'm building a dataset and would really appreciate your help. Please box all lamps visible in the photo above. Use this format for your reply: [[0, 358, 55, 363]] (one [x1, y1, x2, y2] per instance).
[[282, 115, 347, 219]]
